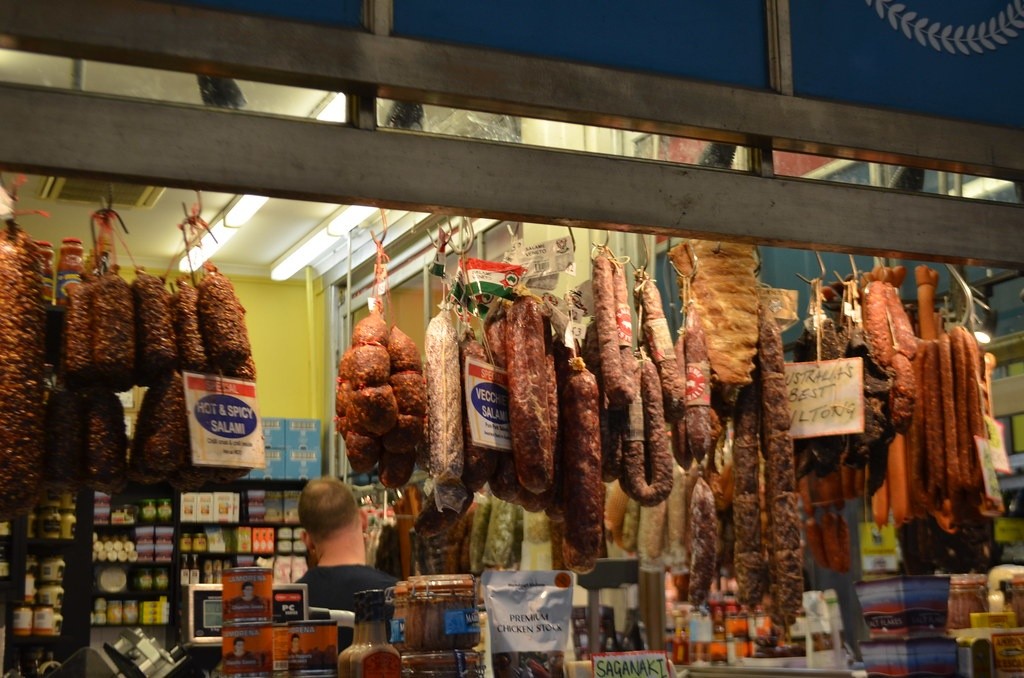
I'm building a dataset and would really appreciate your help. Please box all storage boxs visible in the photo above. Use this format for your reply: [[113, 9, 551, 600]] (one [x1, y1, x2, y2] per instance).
[[854, 573, 960, 678]]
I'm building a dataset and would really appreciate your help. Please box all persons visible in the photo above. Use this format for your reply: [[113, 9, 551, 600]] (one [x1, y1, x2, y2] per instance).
[[294, 476, 399, 613]]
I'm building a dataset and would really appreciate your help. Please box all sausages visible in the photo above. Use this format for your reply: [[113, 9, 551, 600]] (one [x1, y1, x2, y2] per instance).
[[334, 238, 989, 630]]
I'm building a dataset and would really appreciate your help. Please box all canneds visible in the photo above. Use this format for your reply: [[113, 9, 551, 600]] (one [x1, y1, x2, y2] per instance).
[[220, 566, 341, 678], [133, 567, 168, 591], [0, 484, 77, 636], [137, 498, 173, 521], [88, 596, 139, 625], [180, 532, 232, 585]]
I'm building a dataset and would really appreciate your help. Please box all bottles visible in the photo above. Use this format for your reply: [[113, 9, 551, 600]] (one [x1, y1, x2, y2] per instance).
[[338, 590, 401, 678], [180, 554, 200, 585]]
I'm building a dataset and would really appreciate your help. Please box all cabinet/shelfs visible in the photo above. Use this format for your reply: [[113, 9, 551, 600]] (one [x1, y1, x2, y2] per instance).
[[0, 481, 313, 678]]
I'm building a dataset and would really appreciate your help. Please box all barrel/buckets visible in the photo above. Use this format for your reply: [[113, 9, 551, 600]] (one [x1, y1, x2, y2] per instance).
[[220, 567, 338, 678]]
